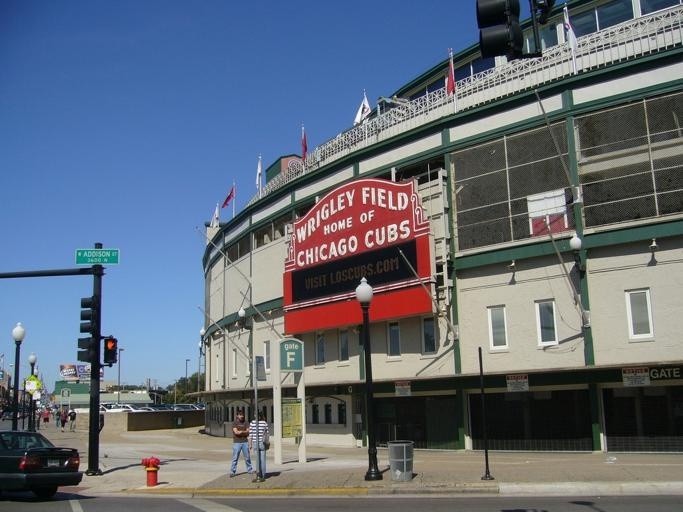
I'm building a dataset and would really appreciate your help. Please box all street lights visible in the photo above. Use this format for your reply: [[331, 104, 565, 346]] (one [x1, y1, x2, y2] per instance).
[[32, 363, 40, 431], [355, 277, 383, 481], [28, 352, 38, 432], [12, 322, 26, 431]]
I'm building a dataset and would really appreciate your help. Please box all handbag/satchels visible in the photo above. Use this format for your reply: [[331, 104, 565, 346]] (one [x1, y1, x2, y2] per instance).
[[263, 428, 270, 450]]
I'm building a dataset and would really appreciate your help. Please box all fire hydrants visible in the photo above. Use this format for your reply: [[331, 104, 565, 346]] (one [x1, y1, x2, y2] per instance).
[[142, 455, 161, 487]]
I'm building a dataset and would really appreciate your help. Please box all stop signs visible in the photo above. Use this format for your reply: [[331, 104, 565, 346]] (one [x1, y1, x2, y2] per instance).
[[103, 338, 119, 365]]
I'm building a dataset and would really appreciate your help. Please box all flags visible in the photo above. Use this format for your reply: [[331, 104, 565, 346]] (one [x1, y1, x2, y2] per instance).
[[299, 129, 308, 162], [209, 202, 218, 229], [446, 56, 455, 96], [256, 158, 262, 191], [221, 185, 234, 209], [561, 7, 579, 54], [351, 91, 372, 127]]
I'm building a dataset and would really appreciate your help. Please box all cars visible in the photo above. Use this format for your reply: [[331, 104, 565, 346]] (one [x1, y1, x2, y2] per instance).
[[2, 411, 34, 420], [1, 431, 84, 497], [99, 402, 206, 413]]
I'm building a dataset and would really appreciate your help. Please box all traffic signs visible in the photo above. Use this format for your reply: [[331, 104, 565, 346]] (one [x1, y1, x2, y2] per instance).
[[74, 248, 120, 265]]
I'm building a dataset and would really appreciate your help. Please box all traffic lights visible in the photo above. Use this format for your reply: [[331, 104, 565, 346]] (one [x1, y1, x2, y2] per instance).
[[78, 296, 94, 362]]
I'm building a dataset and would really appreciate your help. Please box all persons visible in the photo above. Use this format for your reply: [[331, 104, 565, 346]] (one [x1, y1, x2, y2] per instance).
[[248, 407, 270, 483], [34, 404, 77, 432], [228, 409, 256, 477]]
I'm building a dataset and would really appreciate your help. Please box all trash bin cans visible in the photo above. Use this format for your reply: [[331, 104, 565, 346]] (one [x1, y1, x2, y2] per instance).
[[387, 440, 414, 481]]
